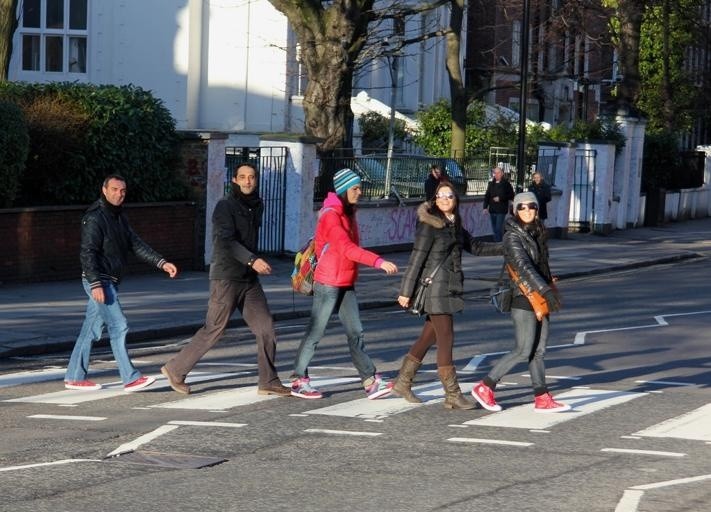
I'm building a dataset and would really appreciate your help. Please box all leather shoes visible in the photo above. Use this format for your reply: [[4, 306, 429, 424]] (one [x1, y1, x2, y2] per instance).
[[257, 383, 291, 397], [160, 363, 190, 394]]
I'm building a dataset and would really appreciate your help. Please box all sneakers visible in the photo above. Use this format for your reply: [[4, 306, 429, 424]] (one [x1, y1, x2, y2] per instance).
[[124, 377, 155, 392], [64, 380, 102, 391], [291, 377, 322, 399], [470, 380, 502, 411], [365, 374, 394, 399], [534, 391, 571, 414]]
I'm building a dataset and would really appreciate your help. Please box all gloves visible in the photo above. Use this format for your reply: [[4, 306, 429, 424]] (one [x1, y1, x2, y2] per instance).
[[543, 291, 560, 313]]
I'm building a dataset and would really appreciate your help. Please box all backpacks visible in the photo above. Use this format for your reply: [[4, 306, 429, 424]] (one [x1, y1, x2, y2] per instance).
[[289, 208, 341, 297]]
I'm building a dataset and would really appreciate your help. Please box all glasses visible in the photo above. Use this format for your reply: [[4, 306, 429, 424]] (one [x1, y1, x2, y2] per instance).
[[517, 202, 538, 210], [435, 192, 455, 200]]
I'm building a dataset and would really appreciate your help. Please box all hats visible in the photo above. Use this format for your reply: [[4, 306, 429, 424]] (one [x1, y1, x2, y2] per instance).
[[513, 191, 539, 221], [333, 168, 361, 195]]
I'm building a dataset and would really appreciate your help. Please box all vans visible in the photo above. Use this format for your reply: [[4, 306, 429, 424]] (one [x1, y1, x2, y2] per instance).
[[354, 153, 468, 197]]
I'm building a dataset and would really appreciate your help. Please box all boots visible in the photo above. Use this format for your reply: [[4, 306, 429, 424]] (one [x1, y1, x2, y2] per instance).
[[438, 366, 481, 410], [391, 354, 422, 403]]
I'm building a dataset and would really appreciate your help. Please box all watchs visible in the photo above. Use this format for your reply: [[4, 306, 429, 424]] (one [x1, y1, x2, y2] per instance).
[[246, 254, 260, 266]]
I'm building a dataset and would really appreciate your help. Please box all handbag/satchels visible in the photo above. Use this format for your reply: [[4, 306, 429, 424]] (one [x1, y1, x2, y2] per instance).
[[490, 282, 512, 313], [527, 277, 562, 321], [405, 275, 431, 315]]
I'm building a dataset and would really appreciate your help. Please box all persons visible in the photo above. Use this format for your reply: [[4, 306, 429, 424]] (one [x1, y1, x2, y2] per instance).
[[528, 172, 553, 227], [469, 191, 573, 414], [390, 181, 505, 411], [62, 172, 179, 391], [290, 168, 399, 399], [482, 167, 514, 242], [160, 162, 292, 396], [425, 164, 442, 201]]
